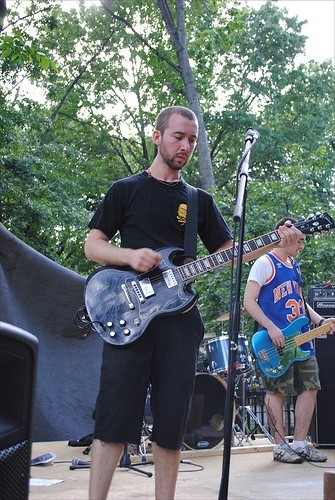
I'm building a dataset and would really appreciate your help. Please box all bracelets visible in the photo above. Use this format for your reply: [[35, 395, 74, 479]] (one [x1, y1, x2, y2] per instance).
[[319, 318, 325, 326]]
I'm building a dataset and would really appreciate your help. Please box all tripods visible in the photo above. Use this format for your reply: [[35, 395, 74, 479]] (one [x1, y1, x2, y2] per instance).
[[69, 444, 155, 476], [231, 319, 275, 446]]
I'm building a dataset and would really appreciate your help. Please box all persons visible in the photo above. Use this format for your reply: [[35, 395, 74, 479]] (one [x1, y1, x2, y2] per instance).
[[243, 217, 335, 464], [84, 104, 303, 500]]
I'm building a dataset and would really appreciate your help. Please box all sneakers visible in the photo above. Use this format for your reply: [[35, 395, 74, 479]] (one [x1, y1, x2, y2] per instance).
[[273, 441, 304, 463], [290, 436, 327, 462]]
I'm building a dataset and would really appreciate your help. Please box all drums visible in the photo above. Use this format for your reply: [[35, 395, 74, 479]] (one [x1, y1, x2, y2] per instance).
[[204, 334, 251, 375], [181, 374, 236, 450]]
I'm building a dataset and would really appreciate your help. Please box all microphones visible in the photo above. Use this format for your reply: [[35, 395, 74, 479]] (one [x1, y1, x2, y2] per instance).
[[253, 130, 260, 143], [72, 457, 90, 465]]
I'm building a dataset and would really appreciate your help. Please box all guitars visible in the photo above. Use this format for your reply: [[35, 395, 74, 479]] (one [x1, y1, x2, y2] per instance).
[[82, 209, 335, 346], [250, 321, 335, 380]]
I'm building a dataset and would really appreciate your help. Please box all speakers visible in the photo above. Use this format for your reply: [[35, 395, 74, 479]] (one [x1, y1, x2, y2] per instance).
[[294, 314, 335, 449], [0, 321, 40, 500]]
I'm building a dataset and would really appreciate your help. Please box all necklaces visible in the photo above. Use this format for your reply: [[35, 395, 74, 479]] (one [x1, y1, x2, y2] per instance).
[[148, 167, 180, 187]]
